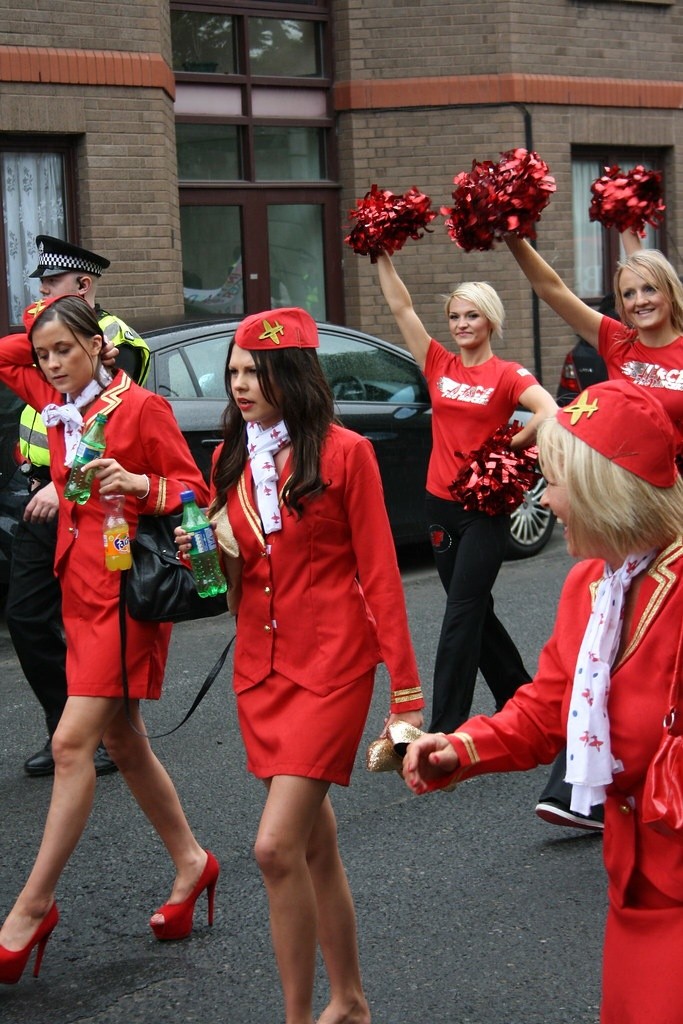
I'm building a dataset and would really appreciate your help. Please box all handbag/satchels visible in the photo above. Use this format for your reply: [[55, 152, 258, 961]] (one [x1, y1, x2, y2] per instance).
[[124, 510, 229, 623]]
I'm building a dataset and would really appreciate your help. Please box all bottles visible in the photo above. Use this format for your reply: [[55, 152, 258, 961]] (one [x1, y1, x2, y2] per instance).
[[98, 484, 134, 572], [64, 412, 108, 505], [180, 491, 228, 597]]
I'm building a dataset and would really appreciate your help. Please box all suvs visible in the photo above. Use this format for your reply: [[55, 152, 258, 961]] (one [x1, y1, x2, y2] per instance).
[[558, 290, 683, 484]]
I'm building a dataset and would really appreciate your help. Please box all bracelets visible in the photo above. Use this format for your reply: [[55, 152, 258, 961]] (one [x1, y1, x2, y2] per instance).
[[137, 474, 150, 500]]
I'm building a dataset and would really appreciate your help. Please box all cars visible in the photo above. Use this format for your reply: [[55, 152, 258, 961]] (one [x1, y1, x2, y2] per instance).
[[0, 312, 556, 612]]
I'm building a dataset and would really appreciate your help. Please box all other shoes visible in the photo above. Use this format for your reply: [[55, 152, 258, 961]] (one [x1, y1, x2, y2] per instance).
[[536, 801, 606, 829]]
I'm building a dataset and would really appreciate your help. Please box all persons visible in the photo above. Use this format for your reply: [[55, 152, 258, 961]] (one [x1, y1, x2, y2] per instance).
[[378, 245, 559, 794], [404, 379, 683, 1024], [0, 234, 153, 777], [503, 227, 683, 831], [174, 307, 425, 1024], [228, 247, 291, 309], [0, 292, 222, 984]]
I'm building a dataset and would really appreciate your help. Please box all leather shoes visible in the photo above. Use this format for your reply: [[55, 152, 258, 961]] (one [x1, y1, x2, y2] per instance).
[[90, 748, 117, 777], [22, 740, 55, 775]]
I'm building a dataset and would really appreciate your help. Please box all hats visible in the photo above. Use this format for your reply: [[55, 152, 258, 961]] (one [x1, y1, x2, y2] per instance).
[[235, 307, 319, 350], [28, 235, 110, 278], [22, 294, 94, 341], [555, 377, 678, 487]]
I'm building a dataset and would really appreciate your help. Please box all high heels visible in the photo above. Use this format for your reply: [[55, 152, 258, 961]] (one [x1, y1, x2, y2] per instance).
[[0, 902, 58, 985], [150, 850, 219, 940], [366, 713, 456, 794]]
